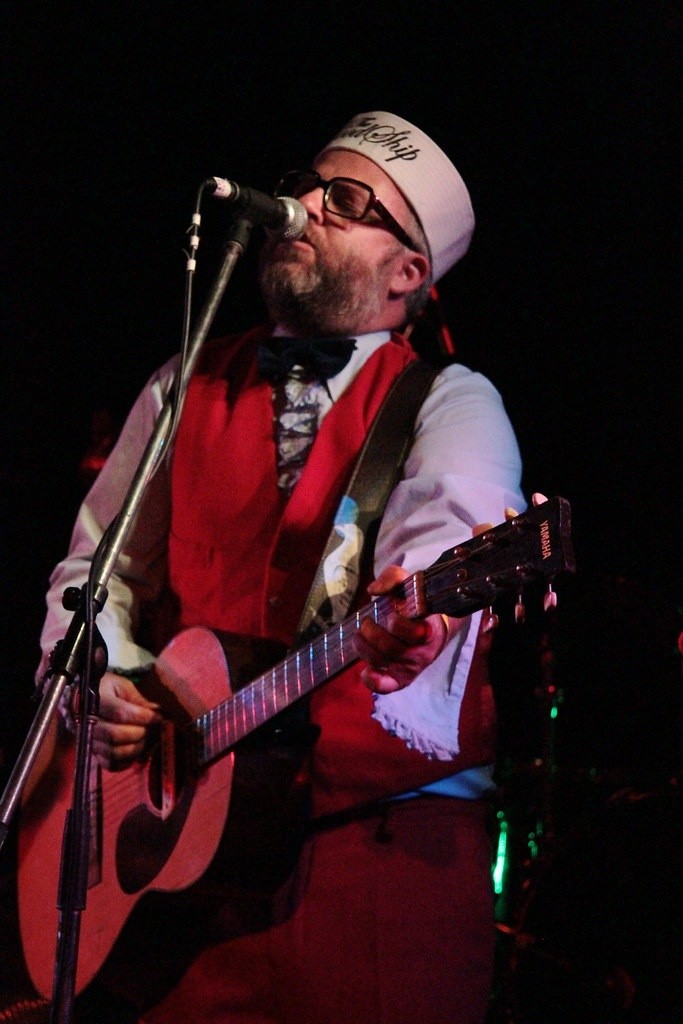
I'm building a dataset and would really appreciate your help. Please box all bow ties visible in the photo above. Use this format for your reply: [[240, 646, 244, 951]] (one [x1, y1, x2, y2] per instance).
[[260, 331, 360, 385]]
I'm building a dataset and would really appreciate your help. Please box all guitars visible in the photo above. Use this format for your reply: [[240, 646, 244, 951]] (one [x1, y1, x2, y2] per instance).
[[18, 492, 586, 1019]]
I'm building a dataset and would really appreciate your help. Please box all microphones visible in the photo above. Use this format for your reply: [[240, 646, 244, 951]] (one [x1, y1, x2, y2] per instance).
[[204, 177, 309, 241]]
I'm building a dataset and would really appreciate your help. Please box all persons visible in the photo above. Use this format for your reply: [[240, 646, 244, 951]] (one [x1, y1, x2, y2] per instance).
[[26, 111, 574, 1022]]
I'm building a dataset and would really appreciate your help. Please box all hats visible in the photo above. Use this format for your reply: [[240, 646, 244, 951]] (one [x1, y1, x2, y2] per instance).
[[308, 108, 474, 286]]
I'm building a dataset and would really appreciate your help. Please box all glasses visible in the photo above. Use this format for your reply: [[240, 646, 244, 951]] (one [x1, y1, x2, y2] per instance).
[[273, 168, 425, 256]]
